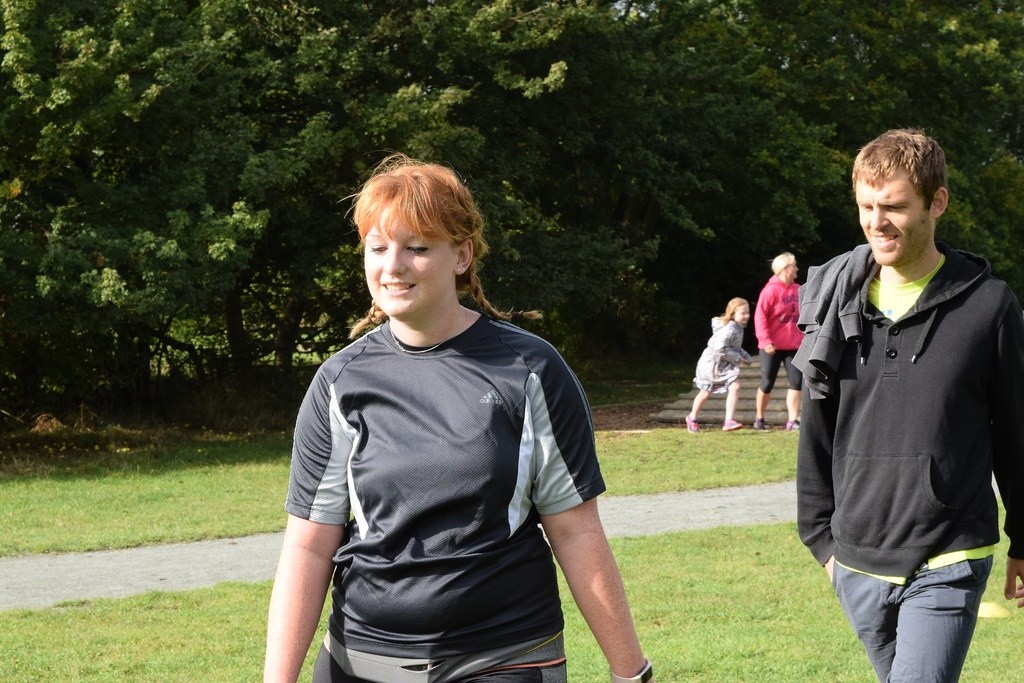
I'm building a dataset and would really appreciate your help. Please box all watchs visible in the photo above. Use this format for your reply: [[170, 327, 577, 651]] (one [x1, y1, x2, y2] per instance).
[[608, 657, 654, 683]]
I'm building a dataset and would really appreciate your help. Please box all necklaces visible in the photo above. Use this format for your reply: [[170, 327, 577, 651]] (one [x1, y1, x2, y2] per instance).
[[388, 313, 482, 355]]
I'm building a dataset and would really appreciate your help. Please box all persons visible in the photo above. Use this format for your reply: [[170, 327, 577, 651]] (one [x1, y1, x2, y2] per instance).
[[261, 164, 657, 683], [792, 124, 1024, 683], [685, 297, 754, 434], [754, 252, 805, 431]]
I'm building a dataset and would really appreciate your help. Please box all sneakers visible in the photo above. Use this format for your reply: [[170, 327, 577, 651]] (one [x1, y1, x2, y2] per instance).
[[685, 415, 700, 432], [722, 419, 744, 430], [753, 418, 767, 430], [785, 420, 800, 430]]
